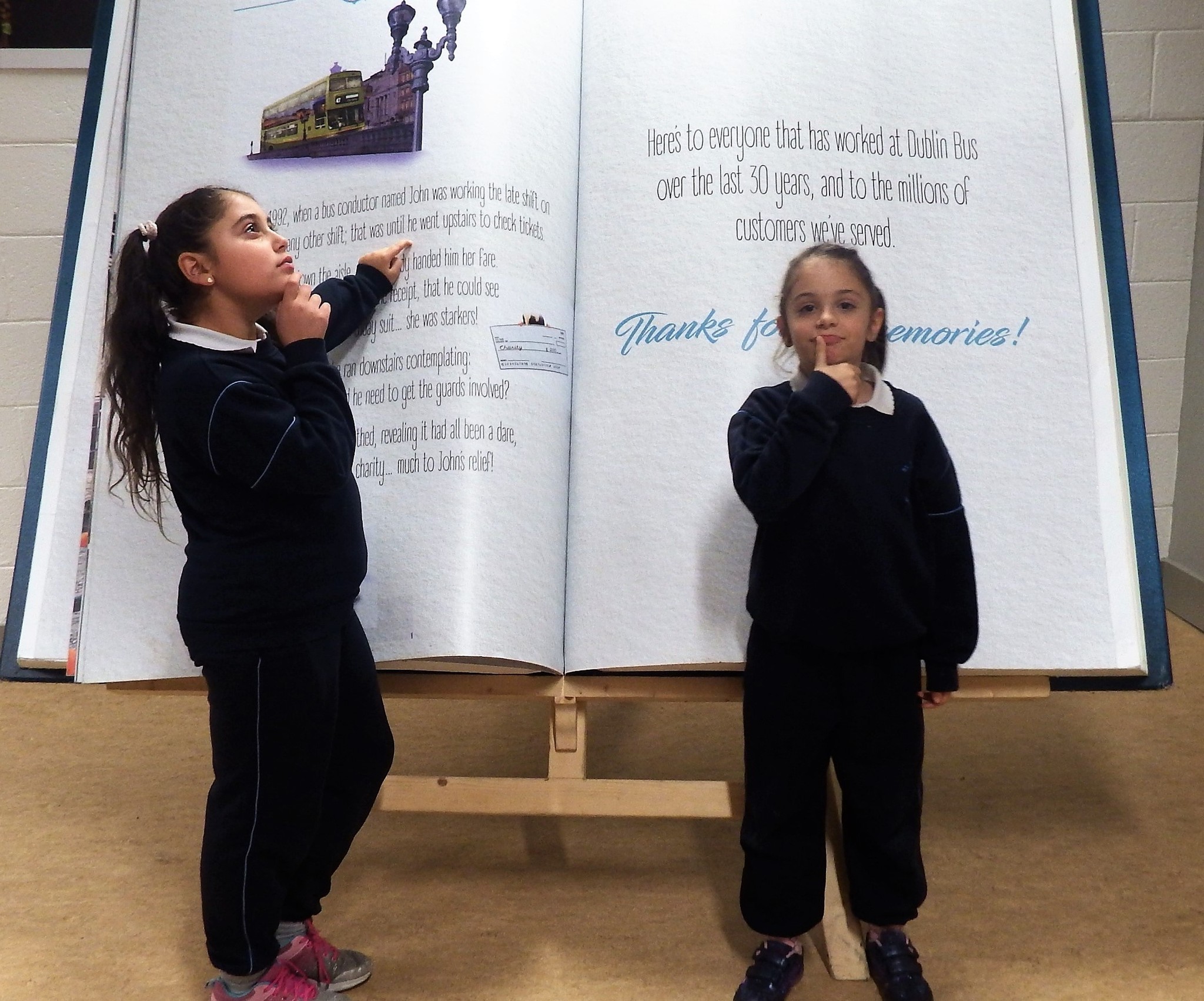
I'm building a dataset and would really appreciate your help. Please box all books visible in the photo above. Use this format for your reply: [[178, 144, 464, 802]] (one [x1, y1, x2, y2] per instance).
[[0, 1, 1175, 690]]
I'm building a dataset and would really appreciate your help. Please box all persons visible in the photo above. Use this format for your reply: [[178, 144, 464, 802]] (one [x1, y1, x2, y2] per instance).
[[101, 186, 412, 1001], [728, 244, 977, 1001]]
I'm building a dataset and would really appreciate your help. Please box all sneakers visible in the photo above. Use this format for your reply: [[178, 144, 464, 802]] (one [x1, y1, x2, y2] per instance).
[[275, 918, 372, 993], [204, 962, 342, 1001], [733, 940, 805, 1001], [865, 927, 934, 1001]]
